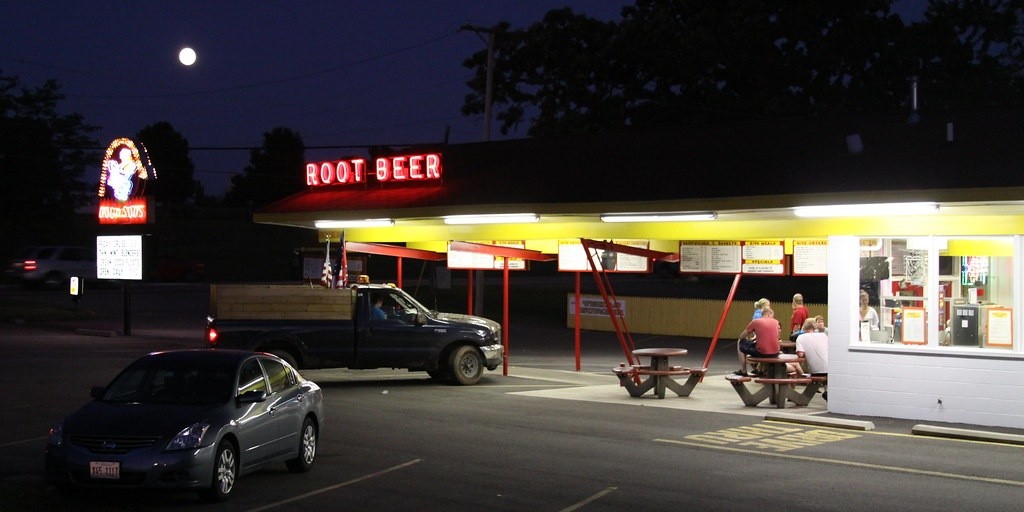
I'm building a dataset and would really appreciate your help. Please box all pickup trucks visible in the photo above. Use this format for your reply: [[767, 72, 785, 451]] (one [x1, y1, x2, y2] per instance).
[[206, 275, 505, 385]]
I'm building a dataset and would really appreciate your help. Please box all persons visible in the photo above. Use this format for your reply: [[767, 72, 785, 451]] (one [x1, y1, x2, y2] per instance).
[[751, 298, 770, 337], [789, 293, 808, 340], [795, 315, 828, 402], [370, 298, 385, 319], [859, 292, 879, 326], [778, 323, 822, 402], [732, 308, 779, 375]]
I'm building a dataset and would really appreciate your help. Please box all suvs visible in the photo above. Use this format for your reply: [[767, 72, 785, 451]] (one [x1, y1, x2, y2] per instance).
[[10, 245, 108, 289]]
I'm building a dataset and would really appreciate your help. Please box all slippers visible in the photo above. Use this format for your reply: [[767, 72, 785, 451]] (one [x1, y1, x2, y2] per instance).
[[734, 369, 748, 375], [752, 370, 758, 374]]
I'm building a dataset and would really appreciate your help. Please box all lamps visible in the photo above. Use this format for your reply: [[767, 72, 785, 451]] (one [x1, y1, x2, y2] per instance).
[[600, 212, 718, 222], [792, 202, 940, 219], [444, 213, 539, 224], [313, 218, 394, 229]]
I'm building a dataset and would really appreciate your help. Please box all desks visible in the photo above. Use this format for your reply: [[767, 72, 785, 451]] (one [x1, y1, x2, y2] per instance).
[[780, 341, 797, 353], [633, 348, 688, 399], [747, 353, 807, 409]]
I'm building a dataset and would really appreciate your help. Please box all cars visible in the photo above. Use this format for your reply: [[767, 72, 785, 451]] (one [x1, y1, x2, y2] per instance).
[[44, 346, 324, 501], [141, 254, 209, 283]]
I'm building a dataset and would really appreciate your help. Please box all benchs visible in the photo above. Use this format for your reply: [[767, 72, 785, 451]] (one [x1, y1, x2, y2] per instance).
[[725, 372, 827, 409], [612, 363, 708, 399]]
